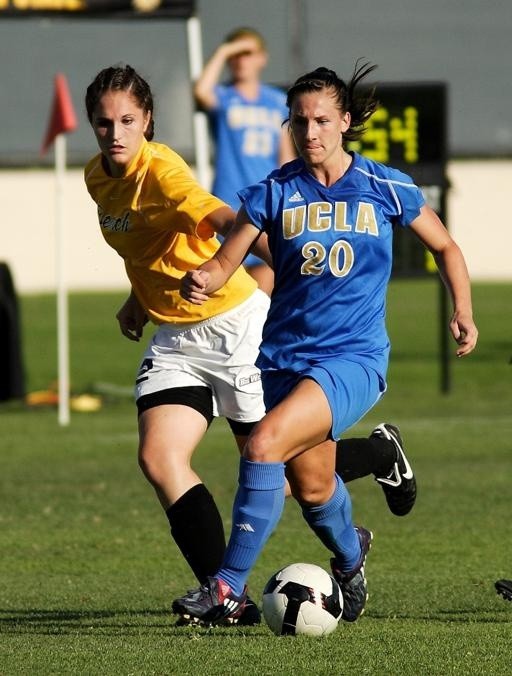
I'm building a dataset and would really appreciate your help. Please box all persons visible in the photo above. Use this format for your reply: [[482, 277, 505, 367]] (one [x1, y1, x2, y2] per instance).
[[192, 28, 298, 299], [170, 55, 478, 624], [84, 64, 417, 628]]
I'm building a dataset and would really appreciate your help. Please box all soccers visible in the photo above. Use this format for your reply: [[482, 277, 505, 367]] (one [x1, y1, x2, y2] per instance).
[[263, 563, 344, 639]]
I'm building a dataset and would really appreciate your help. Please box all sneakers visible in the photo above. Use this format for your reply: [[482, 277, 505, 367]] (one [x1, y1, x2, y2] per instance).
[[172, 576, 261, 627], [370, 423, 417, 517], [329, 525, 373, 623]]
